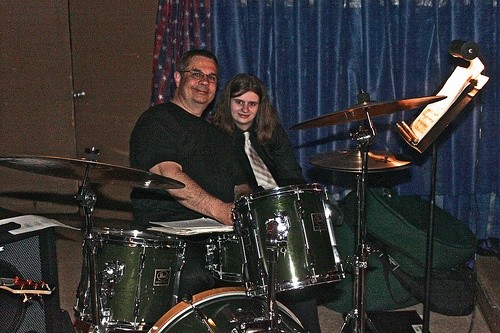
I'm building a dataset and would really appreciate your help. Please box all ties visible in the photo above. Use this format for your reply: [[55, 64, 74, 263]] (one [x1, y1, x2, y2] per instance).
[[243, 131, 280, 191]]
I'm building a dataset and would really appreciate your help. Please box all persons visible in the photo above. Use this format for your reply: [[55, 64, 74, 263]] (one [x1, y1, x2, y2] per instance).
[[213, 73, 308, 192], [130, 49, 251, 230]]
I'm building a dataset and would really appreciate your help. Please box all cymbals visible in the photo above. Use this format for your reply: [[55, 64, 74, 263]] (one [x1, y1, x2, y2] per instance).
[[0, 154, 187, 191], [288, 95, 449, 130], [308, 150, 414, 174]]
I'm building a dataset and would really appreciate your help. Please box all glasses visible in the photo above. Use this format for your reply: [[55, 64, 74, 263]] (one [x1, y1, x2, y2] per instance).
[[182, 68, 219, 83]]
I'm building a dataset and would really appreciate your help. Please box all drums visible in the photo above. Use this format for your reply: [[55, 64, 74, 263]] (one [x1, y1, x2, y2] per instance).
[[73, 226, 187, 332], [204, 232, 246, 284], [232, 182, 346, 295], [147, 286, 310, 333]]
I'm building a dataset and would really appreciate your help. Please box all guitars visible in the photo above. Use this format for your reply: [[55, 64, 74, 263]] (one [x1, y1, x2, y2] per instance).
[[0, 276, 57, 303]]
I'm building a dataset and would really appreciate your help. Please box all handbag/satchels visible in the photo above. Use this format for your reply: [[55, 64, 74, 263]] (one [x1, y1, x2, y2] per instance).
[[318, 187, 479, 317]]
